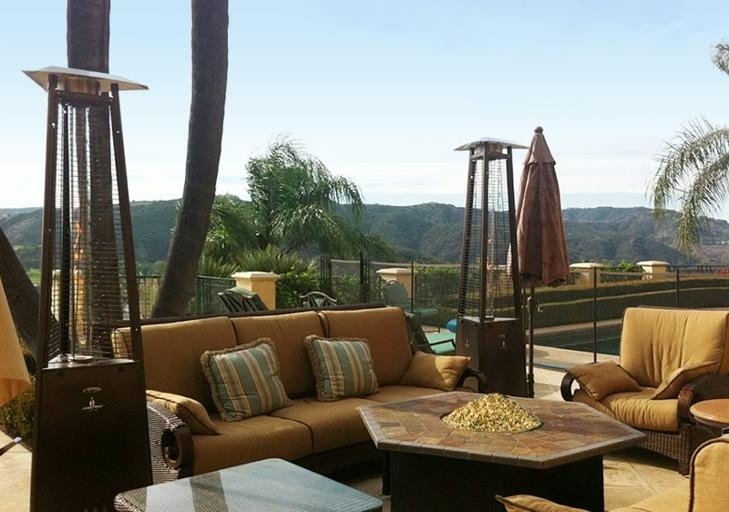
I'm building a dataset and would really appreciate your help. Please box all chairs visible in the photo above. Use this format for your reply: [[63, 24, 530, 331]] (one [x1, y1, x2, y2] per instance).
[[212, 286, 344, 315]]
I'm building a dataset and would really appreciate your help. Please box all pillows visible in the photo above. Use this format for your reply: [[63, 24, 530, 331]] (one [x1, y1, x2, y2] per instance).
[[302, 331, 383, 403], [562, 359, 644, 405], [201, 335, 295, 423], [145, 387, 220, 436], [649, 359, 723, 402], [398, 349, 473, 393], [494, 488, 593, 512]]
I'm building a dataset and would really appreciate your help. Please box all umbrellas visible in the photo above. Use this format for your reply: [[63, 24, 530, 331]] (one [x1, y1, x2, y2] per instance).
[[505, 126, 572, 396]]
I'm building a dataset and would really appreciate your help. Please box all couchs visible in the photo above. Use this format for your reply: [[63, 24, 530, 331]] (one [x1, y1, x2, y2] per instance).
[[494, 435, 729, 512], [557, 303, 729, 477], [89, 304, 492, 487]]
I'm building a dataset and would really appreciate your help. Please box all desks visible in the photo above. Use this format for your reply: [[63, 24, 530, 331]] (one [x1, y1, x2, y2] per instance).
[[689, 396, 729, 436], [112, 455, 386, 512], [350, 389, 648, 512]]
